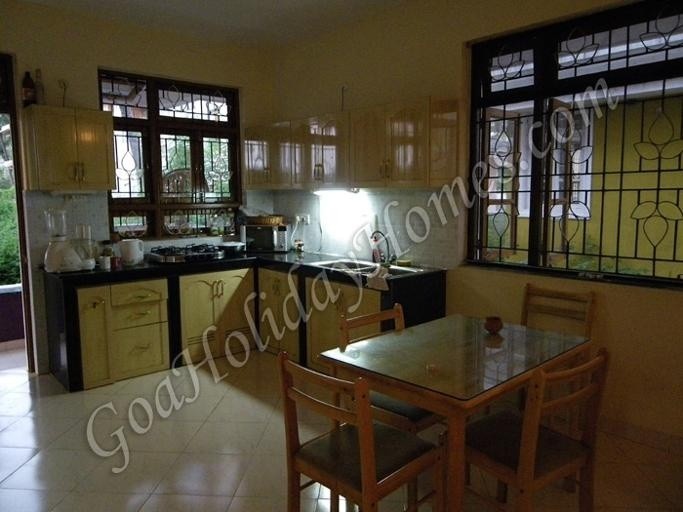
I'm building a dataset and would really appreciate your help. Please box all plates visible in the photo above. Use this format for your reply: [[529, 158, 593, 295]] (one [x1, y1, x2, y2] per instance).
[[395, 260, 411, 266]]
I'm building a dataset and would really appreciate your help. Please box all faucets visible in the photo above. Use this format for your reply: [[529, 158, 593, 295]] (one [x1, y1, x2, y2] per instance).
[[369, 231, 397, 267]]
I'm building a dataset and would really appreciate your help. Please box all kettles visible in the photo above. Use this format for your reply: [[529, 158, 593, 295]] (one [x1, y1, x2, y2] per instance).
[[117, 239, 145, 265]]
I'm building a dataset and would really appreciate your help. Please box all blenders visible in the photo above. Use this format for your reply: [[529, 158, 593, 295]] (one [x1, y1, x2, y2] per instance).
[[41, 208, 72, 272]]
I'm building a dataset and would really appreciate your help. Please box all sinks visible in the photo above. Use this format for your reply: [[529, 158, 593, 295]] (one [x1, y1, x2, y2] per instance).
[[347, 266, 424, 280], [303, 259, 377, 270]]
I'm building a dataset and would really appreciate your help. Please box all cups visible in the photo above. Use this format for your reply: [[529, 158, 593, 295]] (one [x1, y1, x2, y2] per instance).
[[484, 317, 503, 333], [111, 257, 120, 268], [98, 256, 111, 270], [217, 225, 224, 236]]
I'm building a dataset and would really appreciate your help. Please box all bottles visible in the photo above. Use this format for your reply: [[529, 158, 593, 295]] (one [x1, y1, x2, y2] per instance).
[[296, 242, 304, 252], [33, 69, 45, 104], [295, 239, 302, 251], [64, 239, 94, 271], [21, 71, 34, 105], [371, 237, 379, 262]]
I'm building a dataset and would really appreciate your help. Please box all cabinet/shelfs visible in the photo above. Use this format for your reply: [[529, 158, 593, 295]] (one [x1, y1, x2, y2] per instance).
[[23, 103, 116, 193], [240, 94, 467, 191], [41, 256, 447, 393]]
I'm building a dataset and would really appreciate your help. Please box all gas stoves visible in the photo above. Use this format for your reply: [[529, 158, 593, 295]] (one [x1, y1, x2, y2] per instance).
[[149, 242, 223, 262]]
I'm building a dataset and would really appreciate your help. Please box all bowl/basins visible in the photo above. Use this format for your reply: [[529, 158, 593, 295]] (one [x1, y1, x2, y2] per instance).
[[216, 245, 244, 255]]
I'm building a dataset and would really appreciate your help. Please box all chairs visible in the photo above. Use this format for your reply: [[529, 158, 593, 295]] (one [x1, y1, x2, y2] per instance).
[[276, 282, 608, 512]]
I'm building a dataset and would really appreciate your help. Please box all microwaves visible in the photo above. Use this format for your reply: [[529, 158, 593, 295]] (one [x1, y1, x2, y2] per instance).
[[239, 225, 291, 253]]
